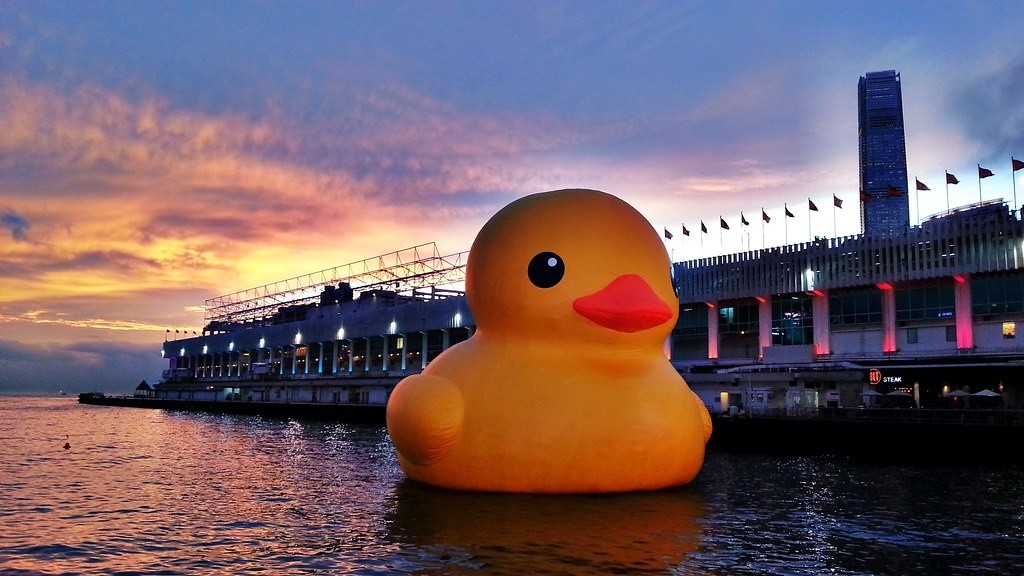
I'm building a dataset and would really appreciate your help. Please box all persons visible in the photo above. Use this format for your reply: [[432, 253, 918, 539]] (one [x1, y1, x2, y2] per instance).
[[724, 408, 730, 416]]
